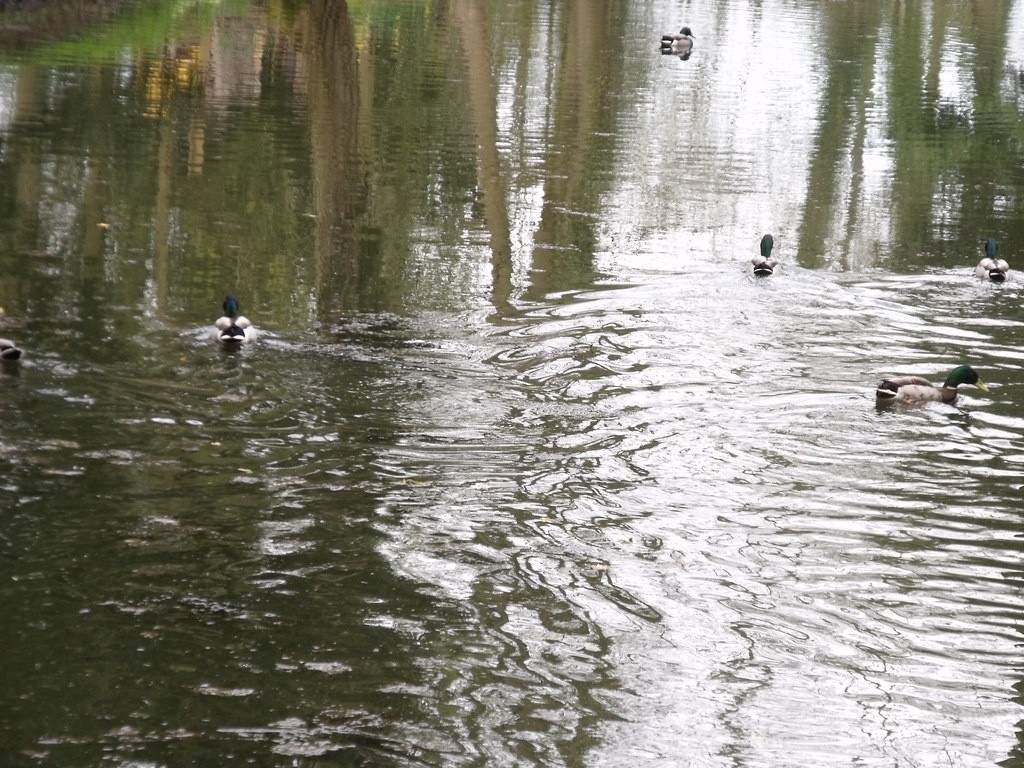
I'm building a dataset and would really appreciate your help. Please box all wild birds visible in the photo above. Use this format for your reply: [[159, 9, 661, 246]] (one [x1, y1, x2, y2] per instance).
[[876, 365, 990, 404], [0, 308, 23, 360], [974, 238, 1009, 282], [214, 296, 251, 341], [661, 27, 695, 45], [751, 234, 780, 274]]
